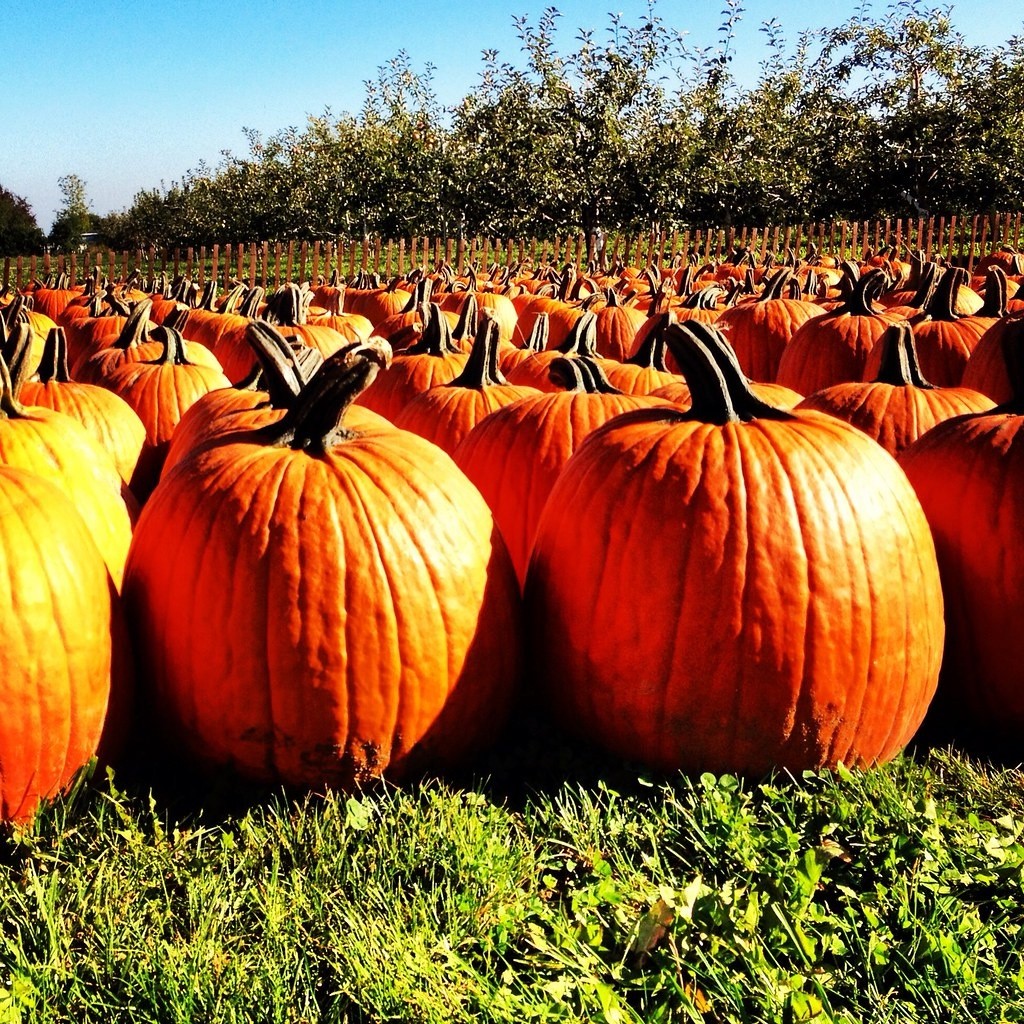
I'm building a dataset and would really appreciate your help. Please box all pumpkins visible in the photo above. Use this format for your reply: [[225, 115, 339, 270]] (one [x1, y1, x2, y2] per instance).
[[0, 242, 1024, 832]]
